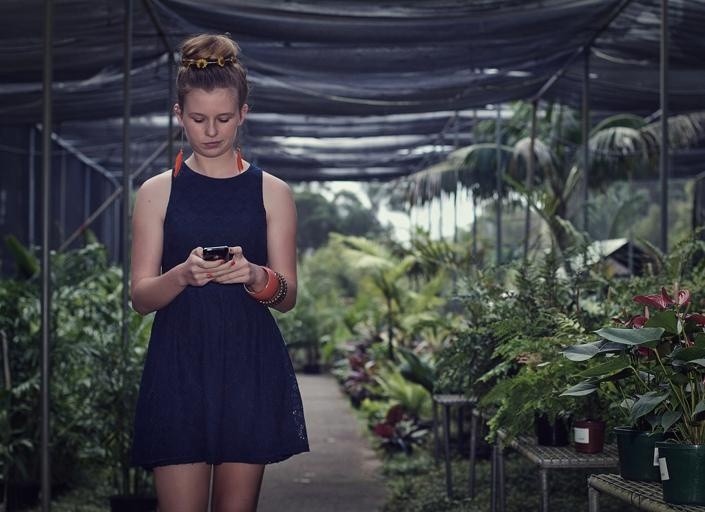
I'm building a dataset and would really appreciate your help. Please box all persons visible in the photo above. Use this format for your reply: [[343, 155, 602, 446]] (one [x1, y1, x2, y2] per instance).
[[129, 35, 310, 512]]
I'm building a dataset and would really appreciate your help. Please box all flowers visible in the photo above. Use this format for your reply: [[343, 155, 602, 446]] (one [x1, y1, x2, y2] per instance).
[[560, 286, 705, 444]]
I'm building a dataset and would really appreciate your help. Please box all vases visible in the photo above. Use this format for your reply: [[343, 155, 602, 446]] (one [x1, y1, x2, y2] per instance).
[[614, 426, 679, 481], [656, 442, 705, 504]]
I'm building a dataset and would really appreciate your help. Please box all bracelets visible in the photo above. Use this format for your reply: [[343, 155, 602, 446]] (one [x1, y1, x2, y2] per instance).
[[260, 270, 289, 308], [242, 265, 279, 301]]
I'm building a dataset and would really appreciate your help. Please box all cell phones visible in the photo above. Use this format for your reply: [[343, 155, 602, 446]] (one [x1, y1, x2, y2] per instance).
[[203, 246, 229, 264]]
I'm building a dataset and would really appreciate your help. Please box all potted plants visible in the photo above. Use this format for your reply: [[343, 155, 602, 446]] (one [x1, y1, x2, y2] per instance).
[[336, 231, 606, 511]]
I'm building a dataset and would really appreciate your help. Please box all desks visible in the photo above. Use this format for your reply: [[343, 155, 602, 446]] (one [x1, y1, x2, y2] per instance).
[[587, 468, 705, 511]]
